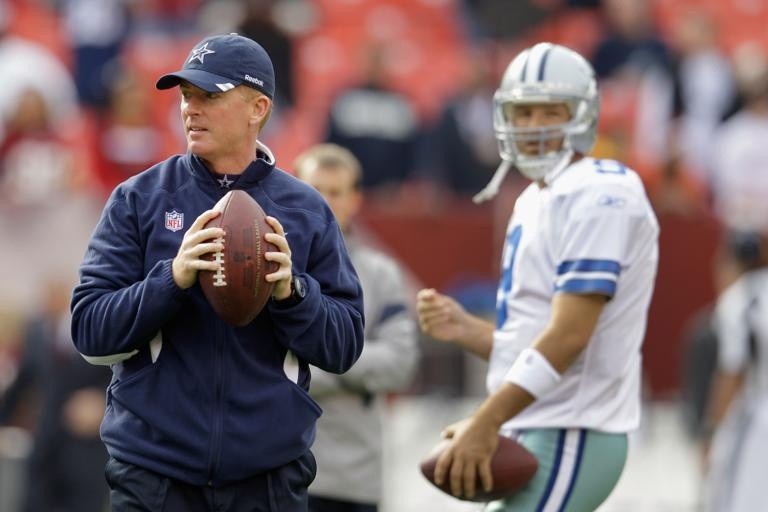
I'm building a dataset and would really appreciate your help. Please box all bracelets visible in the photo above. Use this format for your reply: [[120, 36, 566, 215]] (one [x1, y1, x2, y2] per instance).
[[695, 419, 718, 448]]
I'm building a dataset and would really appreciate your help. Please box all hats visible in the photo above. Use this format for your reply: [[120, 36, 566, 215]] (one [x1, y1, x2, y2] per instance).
[[155, 33, 276, 99]]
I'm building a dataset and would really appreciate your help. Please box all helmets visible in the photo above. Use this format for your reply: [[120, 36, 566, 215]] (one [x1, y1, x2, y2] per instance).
[[492, 42, 600, 152]]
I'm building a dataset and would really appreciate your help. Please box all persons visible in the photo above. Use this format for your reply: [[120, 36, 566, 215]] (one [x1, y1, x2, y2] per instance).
[[70, 30, 366, 508], [693, 215, 766, 511], [282, 143, 422, 511], [677, 226, 759, 461], [415, 41, 662, 512], [0, 258, 112, 511]]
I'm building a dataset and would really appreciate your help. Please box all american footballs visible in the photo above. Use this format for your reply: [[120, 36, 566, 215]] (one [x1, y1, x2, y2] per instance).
[[199, 190, 280, 326], [421, 434, 539, 502]]
